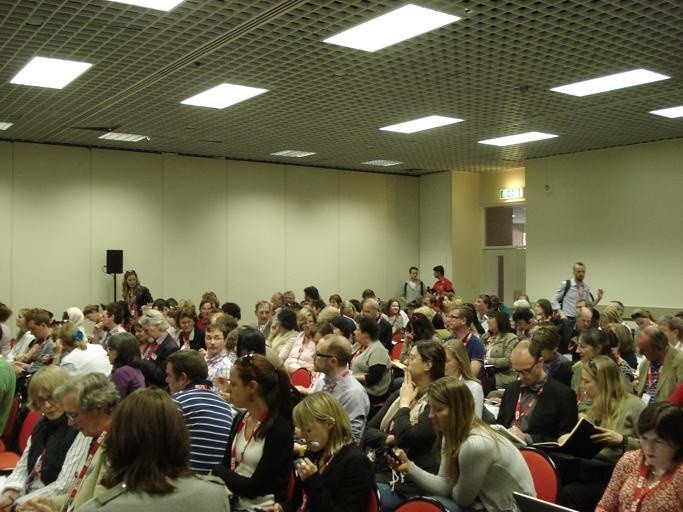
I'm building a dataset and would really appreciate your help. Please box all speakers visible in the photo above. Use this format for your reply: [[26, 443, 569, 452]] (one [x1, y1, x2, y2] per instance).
[[107, 250, 123, 274]]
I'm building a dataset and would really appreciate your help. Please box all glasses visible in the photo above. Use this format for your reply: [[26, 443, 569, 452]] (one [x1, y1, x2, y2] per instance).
[[588, 360, 598, 376], [36, 396, 54, 408], [514, 359, 538, 375], [242, 352, 260, 378], [317, 351, 339, 360], [448, 315, 463, 320]]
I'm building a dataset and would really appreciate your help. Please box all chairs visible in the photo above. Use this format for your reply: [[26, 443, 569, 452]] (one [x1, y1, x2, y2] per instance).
[[0, 332, 683, 512]]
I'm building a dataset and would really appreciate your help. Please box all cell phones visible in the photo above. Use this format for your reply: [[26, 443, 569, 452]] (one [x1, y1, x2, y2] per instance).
[[384, 447, 402, 465]]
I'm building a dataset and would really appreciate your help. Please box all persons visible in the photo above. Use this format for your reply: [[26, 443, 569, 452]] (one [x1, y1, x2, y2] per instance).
[[120, 269, 153, 318], [427, 265, 454, 304], [1, 290, 682, 511], [300, 286, 325, 311], [401, 266, 429, 306], [549, 260, 604, 320]]
